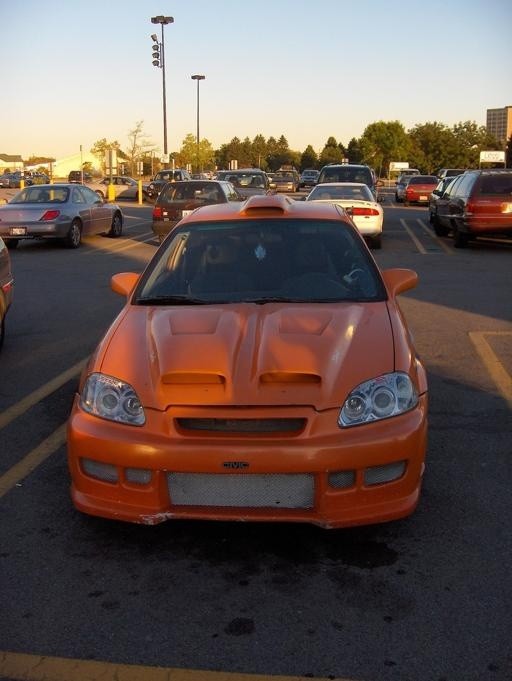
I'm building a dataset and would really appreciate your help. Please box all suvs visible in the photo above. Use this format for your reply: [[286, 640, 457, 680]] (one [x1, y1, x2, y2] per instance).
[[430, 167, 512, 247]]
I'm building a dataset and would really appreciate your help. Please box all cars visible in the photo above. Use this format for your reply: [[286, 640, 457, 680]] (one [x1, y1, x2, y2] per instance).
[[427, 173, 457, 223], [63, 190, 437, 531], [0, 181, 125, 248], [0, 234, 18, 351], [302, 180, 386, 251], [150, 178, 246, 246]]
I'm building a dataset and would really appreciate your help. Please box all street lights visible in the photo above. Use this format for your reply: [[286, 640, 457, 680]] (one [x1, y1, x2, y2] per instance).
[[148, 13, 176, 166], [189, 73, 206, 172]]
